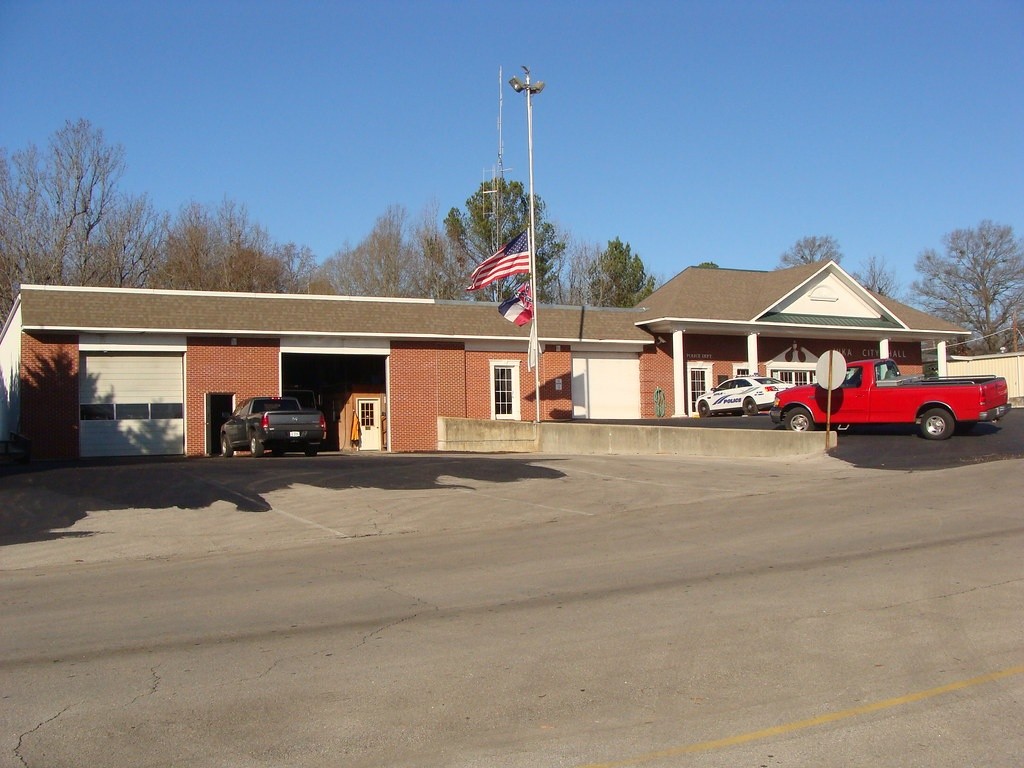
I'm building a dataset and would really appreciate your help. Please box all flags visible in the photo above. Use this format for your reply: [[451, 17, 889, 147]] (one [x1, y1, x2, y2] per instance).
[[497, 282, 536, 326], [522, 318, 542, 373], [466, 225, 534, 292]]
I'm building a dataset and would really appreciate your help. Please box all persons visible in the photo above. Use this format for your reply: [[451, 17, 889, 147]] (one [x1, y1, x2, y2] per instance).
[[854, 372, 862, 387]]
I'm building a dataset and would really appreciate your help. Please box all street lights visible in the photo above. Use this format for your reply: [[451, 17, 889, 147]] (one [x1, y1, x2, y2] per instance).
[[507, 65, 545, 424]]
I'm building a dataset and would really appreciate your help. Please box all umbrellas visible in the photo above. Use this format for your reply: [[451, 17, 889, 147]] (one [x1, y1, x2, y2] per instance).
[[352, 409, 360, 453]]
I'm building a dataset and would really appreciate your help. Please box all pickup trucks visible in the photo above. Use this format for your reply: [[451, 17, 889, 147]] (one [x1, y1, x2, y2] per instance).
[[219, 396, 327, 458], [769, 359, 1012, 440]]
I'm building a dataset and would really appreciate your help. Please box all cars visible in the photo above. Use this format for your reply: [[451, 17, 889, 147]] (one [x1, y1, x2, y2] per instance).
[[694, 376, 797, 418]]
